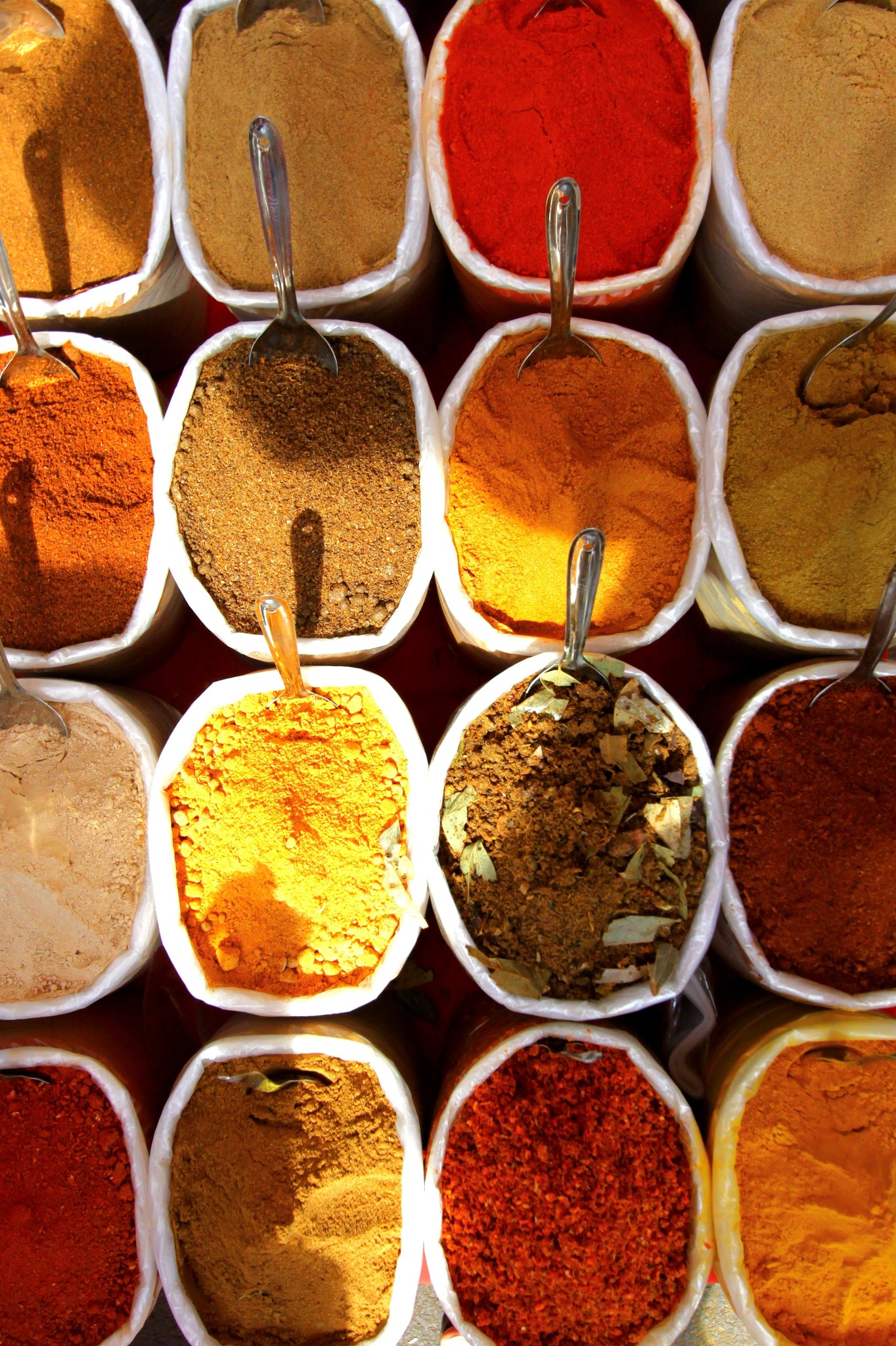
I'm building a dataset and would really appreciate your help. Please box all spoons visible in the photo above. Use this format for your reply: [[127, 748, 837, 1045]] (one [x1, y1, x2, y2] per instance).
[[2, 5, 895, 1108]]
